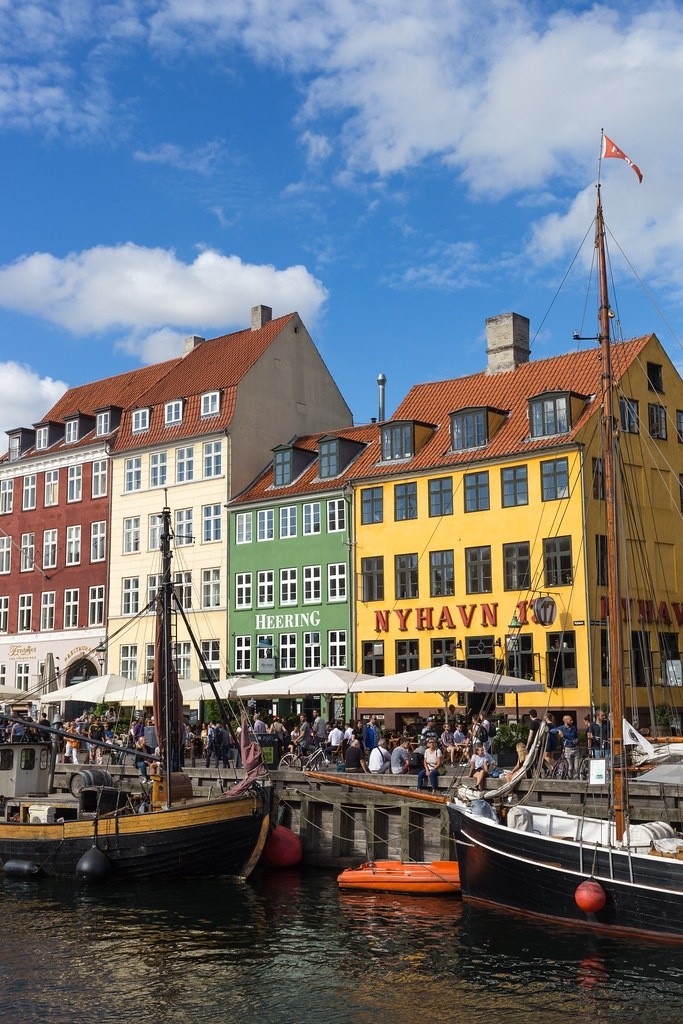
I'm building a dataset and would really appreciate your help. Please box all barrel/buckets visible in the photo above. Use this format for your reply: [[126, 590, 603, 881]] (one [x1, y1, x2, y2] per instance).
[[68, 770, 113, 799]]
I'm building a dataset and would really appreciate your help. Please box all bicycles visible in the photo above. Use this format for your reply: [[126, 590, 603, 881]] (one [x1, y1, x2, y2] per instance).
[[540, 737, 613, 784], [278, 741, 337, 772]]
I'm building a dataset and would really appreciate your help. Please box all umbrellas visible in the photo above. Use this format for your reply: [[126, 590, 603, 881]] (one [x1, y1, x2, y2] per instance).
[[347, 663, 545, 723], [0, 684, 39, 701], [40, 652, 59, 724], [40, 666, 377, 724]]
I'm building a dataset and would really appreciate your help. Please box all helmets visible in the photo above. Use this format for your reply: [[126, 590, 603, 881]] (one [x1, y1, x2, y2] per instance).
[[427, 717, 436, 722]]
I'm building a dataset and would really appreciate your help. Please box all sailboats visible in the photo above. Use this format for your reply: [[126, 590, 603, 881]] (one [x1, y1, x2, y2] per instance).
[[446, 127, 683, 943], [0, 488, 274, 886]]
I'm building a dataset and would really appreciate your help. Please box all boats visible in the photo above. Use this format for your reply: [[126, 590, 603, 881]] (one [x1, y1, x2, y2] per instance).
[[332, 858, 463, 895]]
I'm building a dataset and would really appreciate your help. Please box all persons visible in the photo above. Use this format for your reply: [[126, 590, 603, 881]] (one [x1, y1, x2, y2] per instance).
[[550, 716, 581, 780], [506, 742, 531, 798], [469, 746, 488, 791], [543, 713, 558, 770], [345, 704, 495, 774], [0, 713, 38, 743], [417, 737, 443, 794], [38, 709, 344, 783], [583, 710, 613, 769], [525, 709, 549, 779]]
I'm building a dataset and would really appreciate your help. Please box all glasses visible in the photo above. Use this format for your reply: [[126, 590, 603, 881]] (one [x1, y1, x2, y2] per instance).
[[429, 742, 433, 744]]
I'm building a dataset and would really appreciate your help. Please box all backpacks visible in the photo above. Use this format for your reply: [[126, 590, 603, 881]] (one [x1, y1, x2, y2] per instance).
[[488, 720, 496, 737], [213, 727, 223, 744], [408, 754, 418, 769], [475, 723, 488, 742]]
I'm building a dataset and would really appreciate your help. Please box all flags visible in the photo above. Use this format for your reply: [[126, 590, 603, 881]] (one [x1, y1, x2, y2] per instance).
[[622, 719, 655, 758], [602, 136, 644, 183]]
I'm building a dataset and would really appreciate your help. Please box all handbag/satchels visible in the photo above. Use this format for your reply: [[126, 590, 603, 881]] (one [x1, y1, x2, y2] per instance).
[[437, 765, 447, 776], [383, 757, 387, 763]]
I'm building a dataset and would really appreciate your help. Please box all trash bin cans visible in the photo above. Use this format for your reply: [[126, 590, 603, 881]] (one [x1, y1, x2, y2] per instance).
[[250, 733, 278, 770]]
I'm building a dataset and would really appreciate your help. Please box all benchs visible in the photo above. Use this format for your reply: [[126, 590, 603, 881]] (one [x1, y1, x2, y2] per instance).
[[5, 797, 81, 820]]
